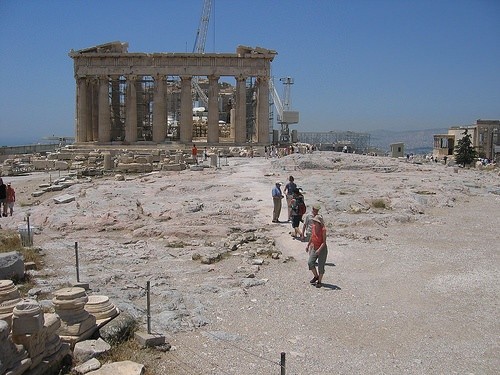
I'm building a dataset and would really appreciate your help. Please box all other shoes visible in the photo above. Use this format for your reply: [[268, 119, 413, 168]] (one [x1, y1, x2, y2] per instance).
[[272, 221, 280, 223], [316, 282, 321, 288], [310, 276, 319, 283]]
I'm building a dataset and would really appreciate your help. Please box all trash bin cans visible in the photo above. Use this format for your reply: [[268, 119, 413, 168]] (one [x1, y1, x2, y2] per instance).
[[19, 225, 34, 248]]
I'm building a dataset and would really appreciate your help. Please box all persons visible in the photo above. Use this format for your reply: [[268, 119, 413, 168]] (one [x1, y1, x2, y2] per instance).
[[272, 181, 283, 222], [306, 214, 327, 288], [193, 144, 198, 165], [301, 203, 323, 265], [0, 178, 7, 217], [6, 182, 15, 216], [283, 176, 297, 221], [265, 143, 315, 159], [203, 146, 208, 161], [288, 189, 303, 238]]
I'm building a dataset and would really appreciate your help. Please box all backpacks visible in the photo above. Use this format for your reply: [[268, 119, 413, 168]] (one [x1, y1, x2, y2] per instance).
[[291, 196, 306, 216]]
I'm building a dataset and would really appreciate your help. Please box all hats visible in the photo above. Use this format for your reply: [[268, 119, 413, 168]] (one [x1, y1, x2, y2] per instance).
[[312, 204, 320, 211], [313, 215, 322, 223], [276, 180, 282, 184], [8, 182, 11, 185]]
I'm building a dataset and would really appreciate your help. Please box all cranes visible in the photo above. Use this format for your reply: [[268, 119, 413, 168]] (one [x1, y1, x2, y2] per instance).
[[269, 73, 295, 147]]
